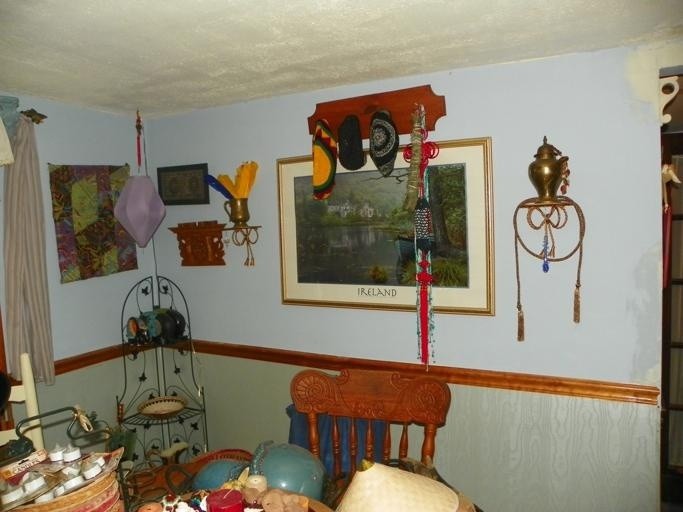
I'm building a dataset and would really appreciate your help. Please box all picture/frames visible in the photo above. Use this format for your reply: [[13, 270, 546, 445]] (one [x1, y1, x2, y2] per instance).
[[155, 162, 210, 205], [278, 134, 498, 316]]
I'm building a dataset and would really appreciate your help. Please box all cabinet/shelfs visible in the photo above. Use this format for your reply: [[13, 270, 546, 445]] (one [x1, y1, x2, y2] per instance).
[[116, 274, 209, 469]]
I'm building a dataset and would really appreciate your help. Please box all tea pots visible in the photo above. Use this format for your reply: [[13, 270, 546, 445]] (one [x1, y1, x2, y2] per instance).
[[526, 134, 568, 201]]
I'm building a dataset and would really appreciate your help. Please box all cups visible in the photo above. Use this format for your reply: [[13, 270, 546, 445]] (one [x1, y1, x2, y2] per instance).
[[223, 197, 249, 229], [297, 496, 308, 512]]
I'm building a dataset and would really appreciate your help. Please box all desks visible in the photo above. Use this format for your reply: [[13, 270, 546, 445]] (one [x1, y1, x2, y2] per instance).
[[152, 487, 338, 511]]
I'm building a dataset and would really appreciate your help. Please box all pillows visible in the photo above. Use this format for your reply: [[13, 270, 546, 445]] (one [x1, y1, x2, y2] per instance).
[[332, 459, 459, 512]]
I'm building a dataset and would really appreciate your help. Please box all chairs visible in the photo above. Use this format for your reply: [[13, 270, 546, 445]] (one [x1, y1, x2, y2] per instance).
[[291, 362, 453, 506]]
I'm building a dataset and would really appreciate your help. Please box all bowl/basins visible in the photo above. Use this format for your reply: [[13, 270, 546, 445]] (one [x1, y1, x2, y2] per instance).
[[137, 396, 187, 419]]
